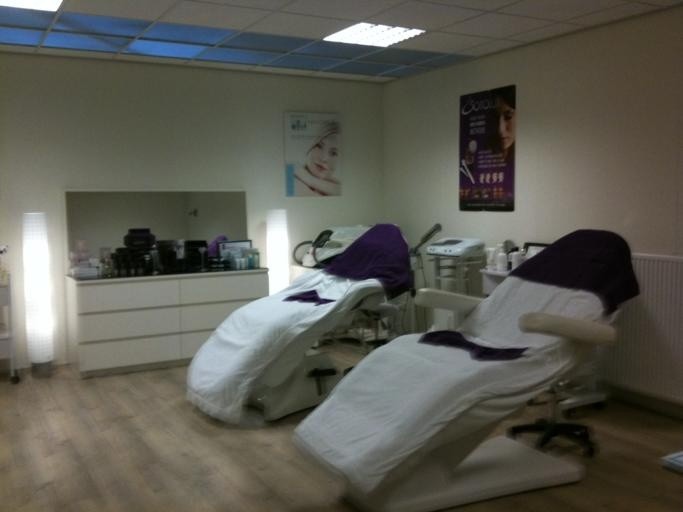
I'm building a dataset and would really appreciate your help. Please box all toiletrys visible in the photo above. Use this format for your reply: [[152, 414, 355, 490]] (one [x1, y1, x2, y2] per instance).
[[496, 246, 523, 272], [232, 246, 261, 270]]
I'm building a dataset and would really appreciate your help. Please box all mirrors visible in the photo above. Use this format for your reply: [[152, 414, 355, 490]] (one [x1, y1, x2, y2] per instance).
[[65, 191, 248, 268]]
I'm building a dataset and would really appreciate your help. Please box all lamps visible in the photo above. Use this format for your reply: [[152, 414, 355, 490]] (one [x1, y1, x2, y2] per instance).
[[21, 212, 56, 378], [266, 209, 292, 298]]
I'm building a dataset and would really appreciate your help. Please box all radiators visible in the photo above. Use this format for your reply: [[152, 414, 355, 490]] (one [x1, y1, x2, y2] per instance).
[[604, 253, 682, 421]]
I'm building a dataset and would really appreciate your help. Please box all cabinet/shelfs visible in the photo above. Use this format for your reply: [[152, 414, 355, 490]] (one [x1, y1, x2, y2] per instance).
[[67, 267, 180, 380], [427, 252, 485, 297], [179, 268, 269, 366]]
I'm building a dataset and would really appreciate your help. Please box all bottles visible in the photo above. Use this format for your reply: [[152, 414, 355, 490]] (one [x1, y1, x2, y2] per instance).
[[511, 247, 521, 270], [498, 243, 509, 271], [233, 249, 259, 270]]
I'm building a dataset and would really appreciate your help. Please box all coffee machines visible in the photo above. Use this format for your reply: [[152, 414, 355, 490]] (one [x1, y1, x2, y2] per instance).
[[123, 228, 156, 276]]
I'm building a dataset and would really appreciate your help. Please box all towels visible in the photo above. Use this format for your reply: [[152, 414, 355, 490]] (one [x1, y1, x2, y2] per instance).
[[314, 223, 416, 299], [419, 330, 529, 361], [282, 290, 336, 307], [506, 229, 639, 316]]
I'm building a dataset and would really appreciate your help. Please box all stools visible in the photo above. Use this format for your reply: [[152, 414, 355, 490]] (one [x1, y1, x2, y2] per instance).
[[362, 303, 401, 351], [507, 343, 605, 459]]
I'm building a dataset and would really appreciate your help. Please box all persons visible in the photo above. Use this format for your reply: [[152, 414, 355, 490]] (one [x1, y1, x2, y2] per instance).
[[492, 97, 517, 209], [293, 119, 343, 196]]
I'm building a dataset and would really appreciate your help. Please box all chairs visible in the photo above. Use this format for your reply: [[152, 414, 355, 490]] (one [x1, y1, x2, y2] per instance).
[[185, 223, 416, 425], [288, 228, 641, 511]]
